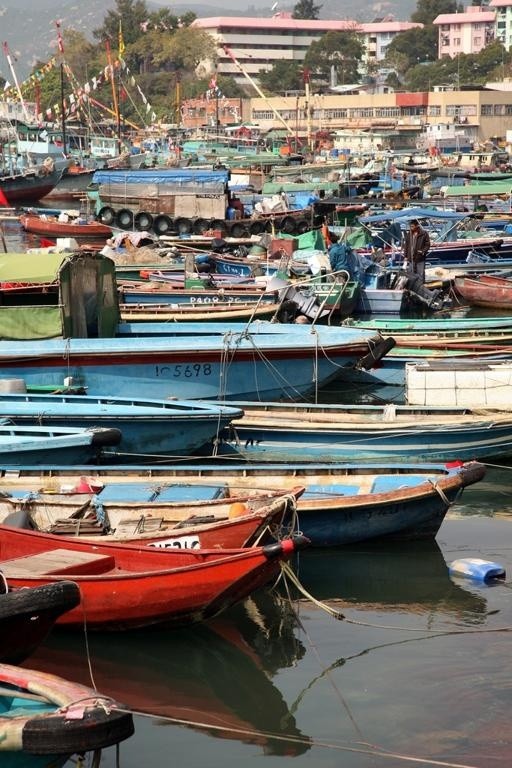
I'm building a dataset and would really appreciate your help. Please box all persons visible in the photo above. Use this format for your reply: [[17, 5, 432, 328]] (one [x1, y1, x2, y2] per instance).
[[404, 219, 430, 283]]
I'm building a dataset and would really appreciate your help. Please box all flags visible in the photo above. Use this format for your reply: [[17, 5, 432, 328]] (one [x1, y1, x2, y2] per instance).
[[1, 30, 182, 158], [211, 79, 231, 111]]
